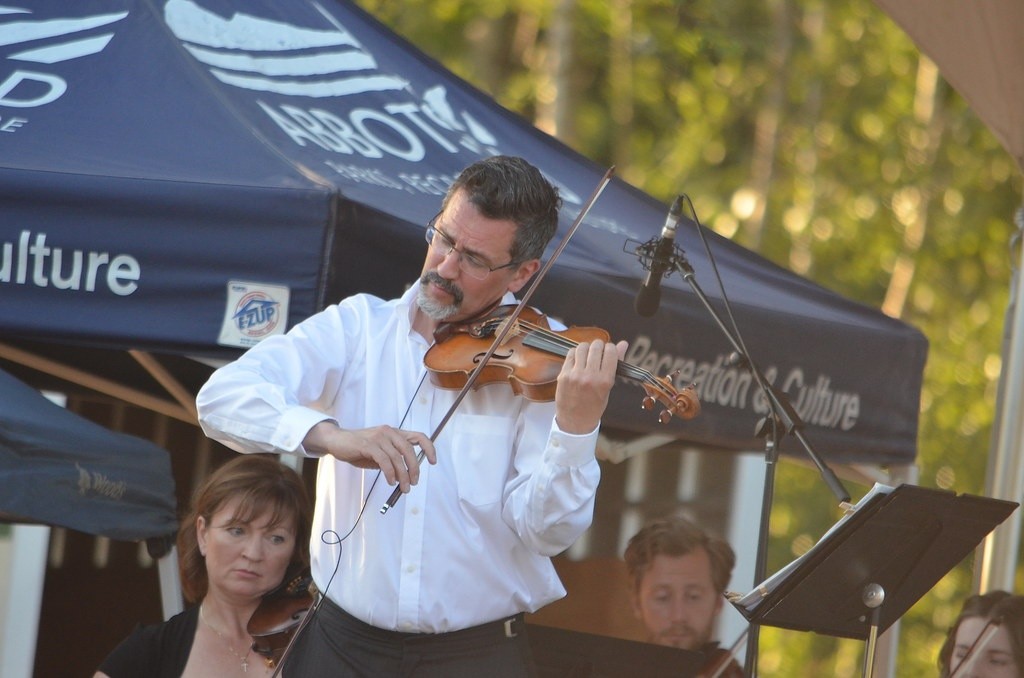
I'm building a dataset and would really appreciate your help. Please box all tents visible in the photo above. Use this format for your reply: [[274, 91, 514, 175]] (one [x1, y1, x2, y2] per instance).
[[0, 0, 928, 677], [0, 370, 185, 624]]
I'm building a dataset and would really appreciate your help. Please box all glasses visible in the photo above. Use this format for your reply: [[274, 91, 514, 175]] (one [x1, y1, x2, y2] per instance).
[[424, 211, 513, 281]]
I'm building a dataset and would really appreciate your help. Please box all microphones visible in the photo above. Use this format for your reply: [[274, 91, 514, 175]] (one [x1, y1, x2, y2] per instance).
[[633, 194, 684, 317]]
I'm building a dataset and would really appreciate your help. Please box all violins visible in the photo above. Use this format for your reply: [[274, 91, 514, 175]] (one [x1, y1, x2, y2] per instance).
[[694, 641, 744, 678], [246, 563, 320, 668], [422, 303, 702, 424]]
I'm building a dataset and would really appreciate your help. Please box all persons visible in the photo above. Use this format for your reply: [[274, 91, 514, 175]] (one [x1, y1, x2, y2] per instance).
[[93, 454, 315, 678], [623, 519, 743, 678], [936, 589, 1024, 678], [195, 157, 630, 677]]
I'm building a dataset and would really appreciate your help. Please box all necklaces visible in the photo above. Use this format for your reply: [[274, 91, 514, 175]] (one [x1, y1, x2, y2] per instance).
[[200, 607, 254, 672]]
